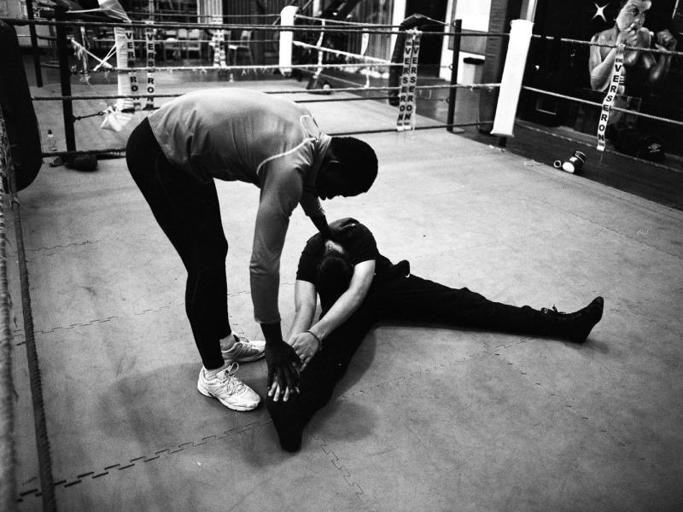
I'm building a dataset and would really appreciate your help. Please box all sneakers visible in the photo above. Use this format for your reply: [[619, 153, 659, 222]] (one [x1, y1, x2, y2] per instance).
[[196, 361, 263, 412], [220, 333, 267, 366]]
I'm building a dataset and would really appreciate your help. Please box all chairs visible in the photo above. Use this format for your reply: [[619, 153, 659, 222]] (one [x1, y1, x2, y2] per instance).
[[177, 29, 201, 59], [227, 30, 254, 65]]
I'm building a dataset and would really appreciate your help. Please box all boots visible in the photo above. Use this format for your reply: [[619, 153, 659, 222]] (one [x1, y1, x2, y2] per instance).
[[265, 369, 325, 452], [537, 297, 603, 344]]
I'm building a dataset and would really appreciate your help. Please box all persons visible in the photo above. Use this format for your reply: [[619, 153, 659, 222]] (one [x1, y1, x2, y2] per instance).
[[122, 86, 379, 413], [587, 0, 679, 157], [264, 215, 605, 453]]
